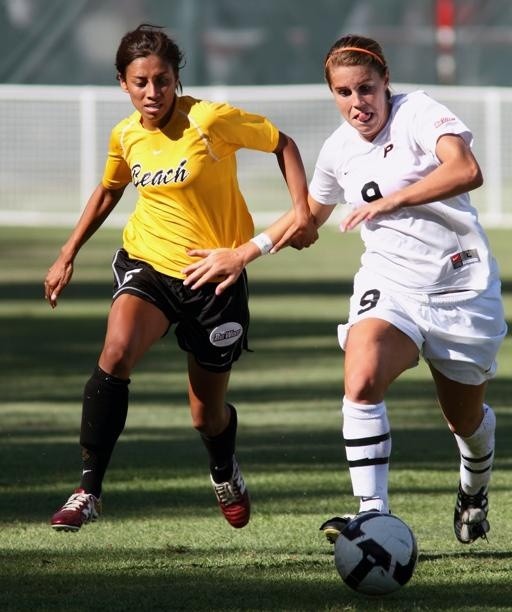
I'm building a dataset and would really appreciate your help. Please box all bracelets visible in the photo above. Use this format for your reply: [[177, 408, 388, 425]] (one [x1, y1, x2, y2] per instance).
[[250, 230, 276, 257]]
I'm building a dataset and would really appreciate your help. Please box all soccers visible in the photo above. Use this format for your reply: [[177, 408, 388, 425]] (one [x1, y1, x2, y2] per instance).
[[335, 511, 417, 596]]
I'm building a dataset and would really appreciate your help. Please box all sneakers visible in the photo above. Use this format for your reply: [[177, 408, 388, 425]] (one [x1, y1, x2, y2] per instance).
[[321, 510, 393, 543], [210, 454, 252, 528], [51, 488, 103, 532], [453, 480, 491, 544]]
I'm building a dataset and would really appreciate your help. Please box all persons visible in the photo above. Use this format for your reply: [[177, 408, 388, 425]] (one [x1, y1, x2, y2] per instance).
[[42, 22, 318, 532], [182, 32, 510, 546]]
